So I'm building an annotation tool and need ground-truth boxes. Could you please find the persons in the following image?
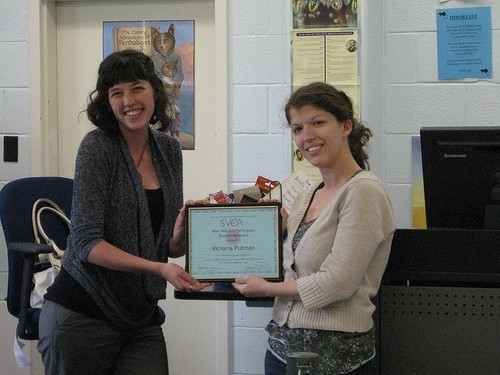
[37,49,213,375]
[234,81,397,375]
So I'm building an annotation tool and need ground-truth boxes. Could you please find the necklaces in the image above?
[133,139,149,168]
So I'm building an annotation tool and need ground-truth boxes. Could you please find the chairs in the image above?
[0,176,74,341]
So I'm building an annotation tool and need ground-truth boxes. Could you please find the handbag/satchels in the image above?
[27,198,73,310]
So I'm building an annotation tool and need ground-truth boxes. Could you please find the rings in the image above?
[189,285,193,291]
